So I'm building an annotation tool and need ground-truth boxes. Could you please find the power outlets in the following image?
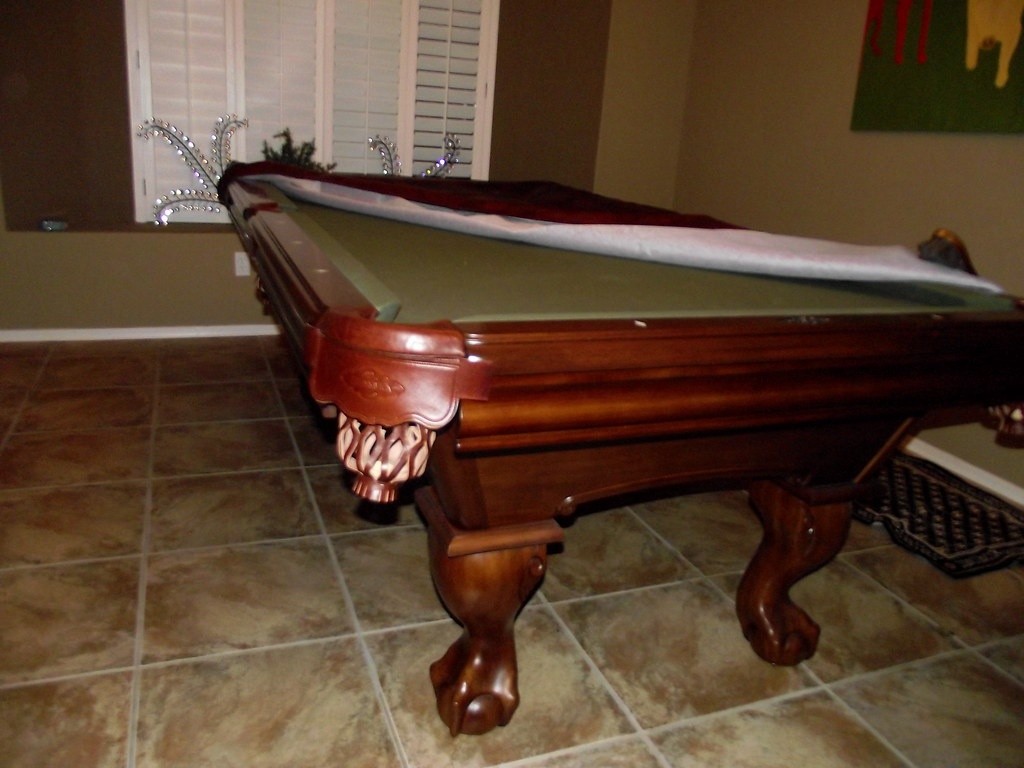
[233,252,252,277]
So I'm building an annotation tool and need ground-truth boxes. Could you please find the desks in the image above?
[215,161,1024,739]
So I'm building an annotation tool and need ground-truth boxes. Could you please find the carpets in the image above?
[847,449,1024,582]
